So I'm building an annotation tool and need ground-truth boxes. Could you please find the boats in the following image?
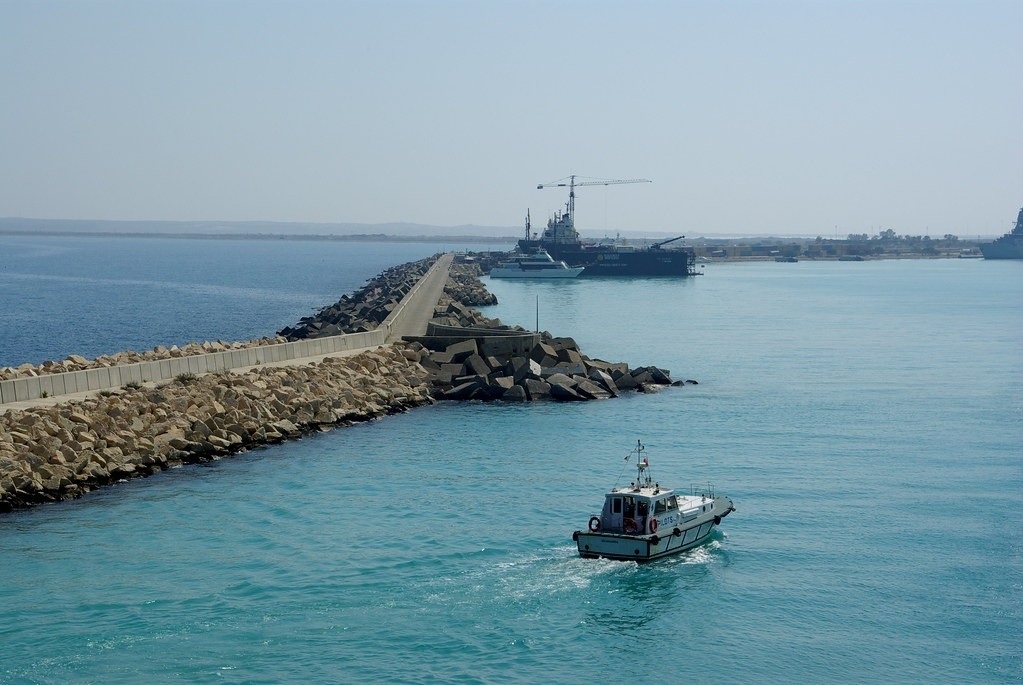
[572,438,738,564]
[518,199,689,276]
[488,207,586,279]
[978,206,1022,260]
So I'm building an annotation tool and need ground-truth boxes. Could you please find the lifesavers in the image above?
[648,517,659,534]
[588,516,601,532]
[622,518,637,534]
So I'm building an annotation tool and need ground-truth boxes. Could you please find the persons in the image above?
[702,494,705,502]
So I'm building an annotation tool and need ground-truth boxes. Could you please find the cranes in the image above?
[536,174,655,227]
